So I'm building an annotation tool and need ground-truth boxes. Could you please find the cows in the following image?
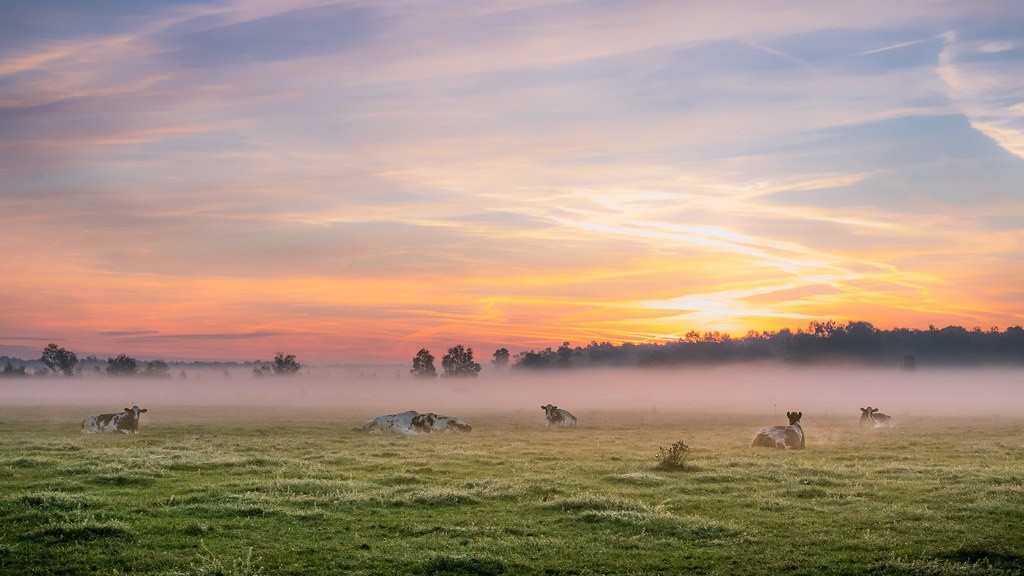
[80,405,148,435]
[541,404,577,427]
[859,407,895,429]
[749,412,806,450]
[352,411,472,437]
